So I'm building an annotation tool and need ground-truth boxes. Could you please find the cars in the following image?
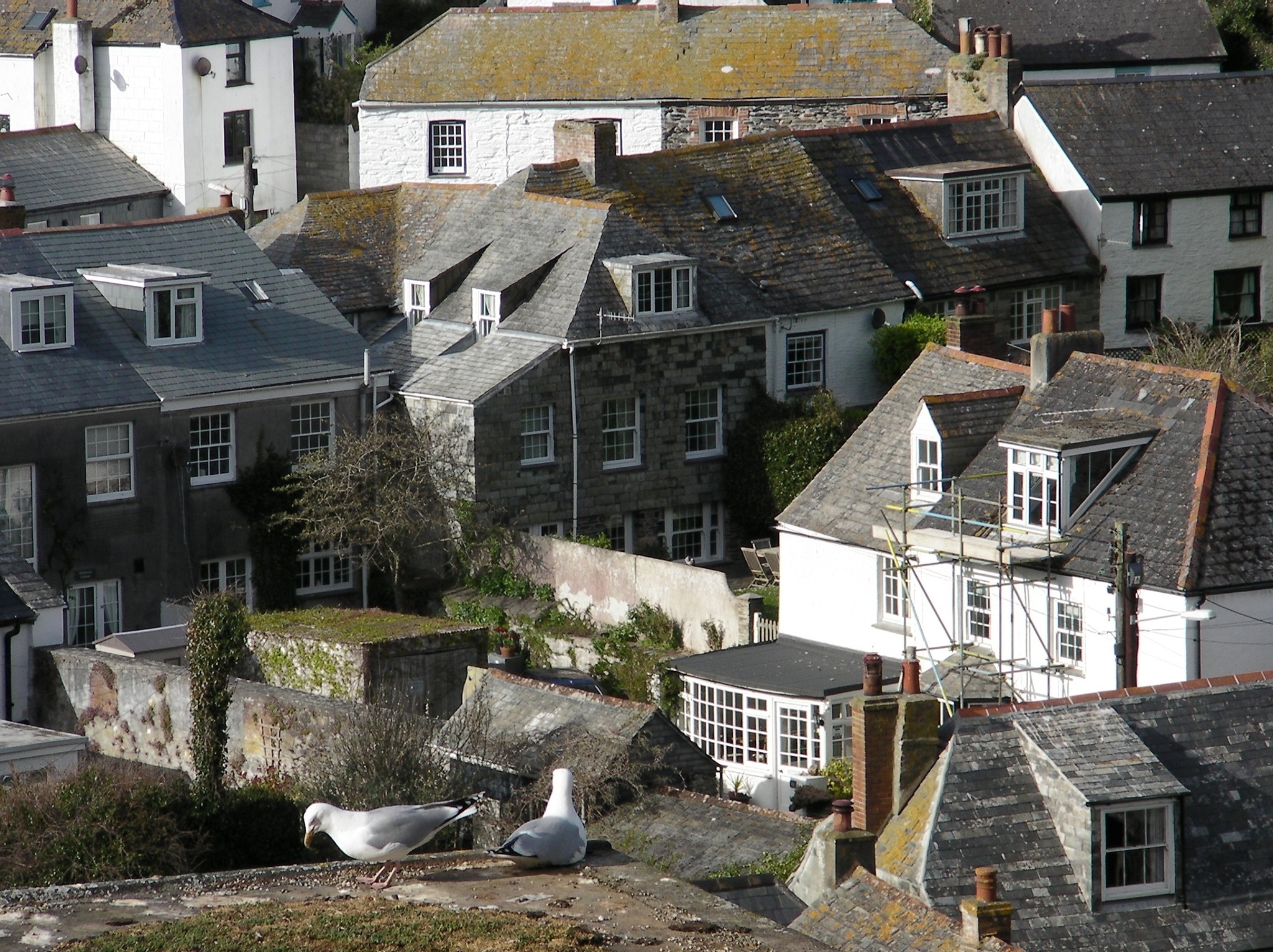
[517,668,605,698]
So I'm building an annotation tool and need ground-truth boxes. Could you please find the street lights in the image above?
[1125,608,1218,688]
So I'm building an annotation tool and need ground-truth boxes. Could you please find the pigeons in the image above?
[492,767,588,868]
[302,795,490,887]
